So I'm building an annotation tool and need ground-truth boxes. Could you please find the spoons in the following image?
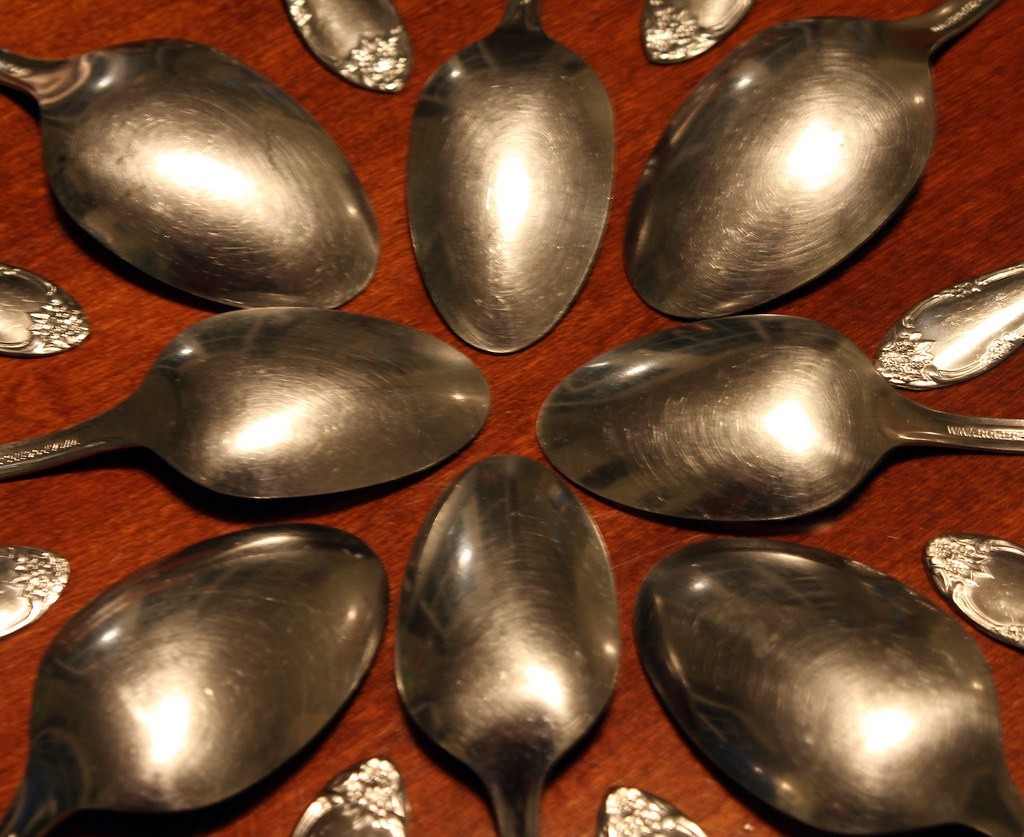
[0,37,382,311]
[403,1,614,356]
[0,306,492,500]
[534,313,1024,523]
[633,535,1024,837]
[625,0,1006,320]
[1,522,387,836]
[393,454,623,837]
